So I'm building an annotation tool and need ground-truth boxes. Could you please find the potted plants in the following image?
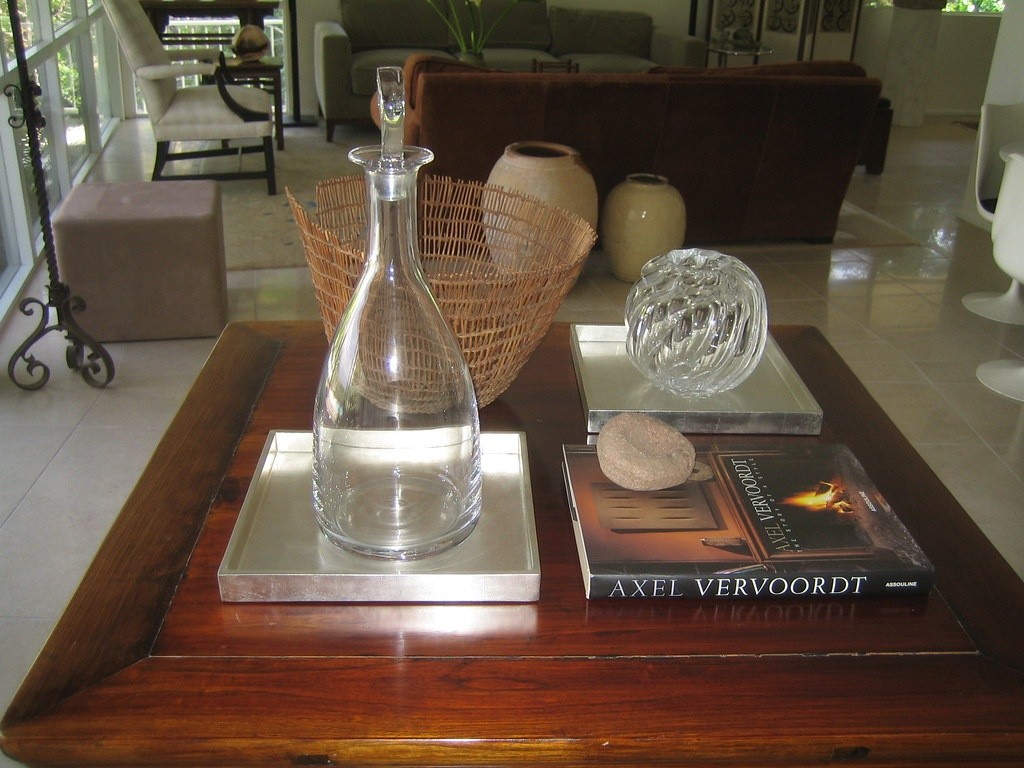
[426,0,518,67]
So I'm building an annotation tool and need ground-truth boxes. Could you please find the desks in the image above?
[707,45,775,68]
[138,0,279,88]
[0,321,1024,768]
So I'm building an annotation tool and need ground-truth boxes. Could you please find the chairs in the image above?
[102,0,277,195]
[961,103,1024,400]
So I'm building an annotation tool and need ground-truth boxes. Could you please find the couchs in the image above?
[314,0,708,153]
[365,54,882,245]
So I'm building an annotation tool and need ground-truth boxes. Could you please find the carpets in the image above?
[146,136,928,269]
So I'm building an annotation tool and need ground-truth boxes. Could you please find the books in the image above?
[561,444,935,600]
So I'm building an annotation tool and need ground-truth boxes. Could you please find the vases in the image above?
[482,139,599,299]
[602,174,686,285]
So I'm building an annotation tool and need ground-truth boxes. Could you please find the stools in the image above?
[48,179,227,345]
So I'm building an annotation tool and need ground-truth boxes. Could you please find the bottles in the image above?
[484,139,601,299]
[599,172,687,286]
[310,66,486,563]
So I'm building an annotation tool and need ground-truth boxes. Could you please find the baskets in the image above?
[284,173,599,414]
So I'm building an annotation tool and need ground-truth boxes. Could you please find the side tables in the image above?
[200,54,286,153]
